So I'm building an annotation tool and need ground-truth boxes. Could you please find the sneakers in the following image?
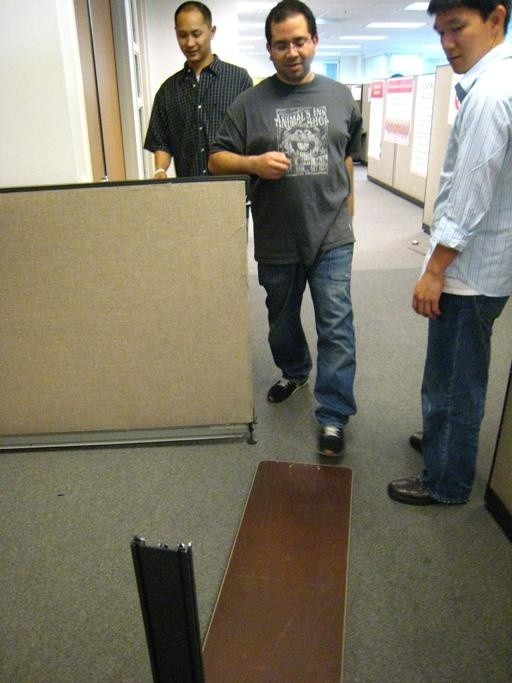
[317,425,344,457]
[267,375,310,403]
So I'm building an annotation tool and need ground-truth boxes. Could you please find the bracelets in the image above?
[153,168,165,176]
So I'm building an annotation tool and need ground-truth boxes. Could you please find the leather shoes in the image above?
[389,477,438,506]
[410,432,424,451]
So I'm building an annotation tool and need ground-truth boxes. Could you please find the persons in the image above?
[206,0,363,458]
[143,0,253,247]
[388,0,510,506]
